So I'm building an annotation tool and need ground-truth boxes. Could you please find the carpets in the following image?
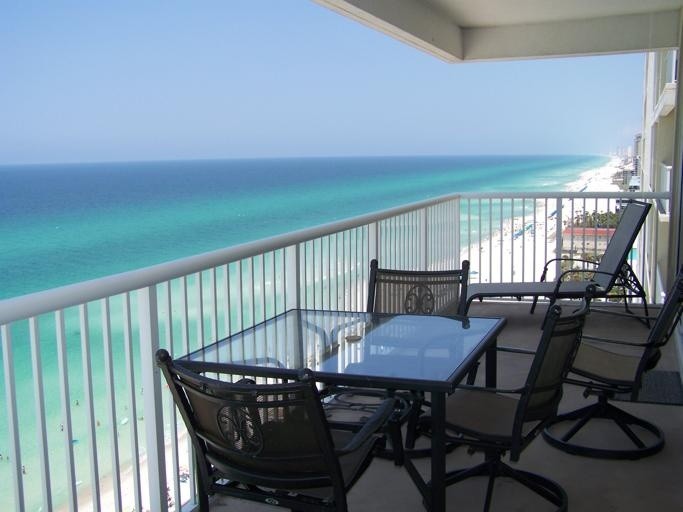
[606,370,681,407]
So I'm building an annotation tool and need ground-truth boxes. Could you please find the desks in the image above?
[182,306,510,511]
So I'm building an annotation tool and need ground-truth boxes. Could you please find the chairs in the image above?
[411,281,595,511]
[460,197,651,327]
[151,339,388,511]
[350,256,471,446]
[543,251,682,458]
